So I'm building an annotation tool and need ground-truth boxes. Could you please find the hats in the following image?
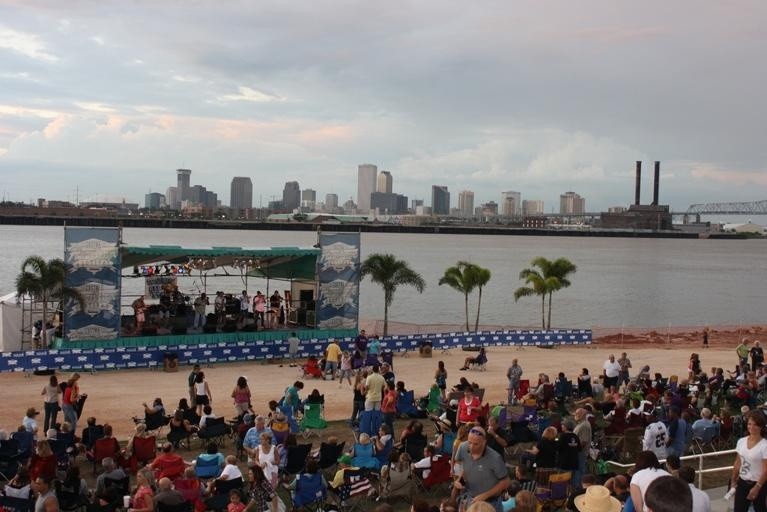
[375,503,394,512]
[46,320,52,329]
[337,455,353,465]
[26,408,40,418]
[273,414,288,424]
[625,408,642,420]
[436,419,452,432]
[257,291,262,294]
[553,433,586,470]
[34,320,41,331]
[638,400,656,416]
[745,371,757,378]
[66,465,82,481]
[271,422,288,433]
[741,405,750,413]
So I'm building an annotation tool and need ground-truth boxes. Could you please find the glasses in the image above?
[470,427,486,440]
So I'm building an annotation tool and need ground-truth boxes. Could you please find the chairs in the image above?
[85,499,119,512]
[158,498,192,511]
[183,405,198,424]
[300,403,324,438]
[10,431,35,455]
[396,390,415,415]
[87,440,120,475]
[434,432,459,454]
[643,402,657,422]
[627,411,642,427]
[229,414,257,433]
[553,379,573,406]
[471,353,487,372]
[354,443,375,460]
[711,417,735,451]
[507,379,530,401]
[377,437,394,459]
[53,480,85,510]
[329,466,370,508]
[533,383,554,408]
[122,435,156,475]
[604,407,626,436]
[306,394,325,404]
[371,471,412,505]
[573,377,591,395]
[133,407,165,436]
[282,472,325,512]
[5,482,33,500]
[687,422,722,455]
[309,440,347,469]
[208,476,243,506]
[392,433,428,458]
[198,417,225,449]
[623,426,642,464]
[194,456,221,478]
[279,442,313,474]
[510,420,530,437]
[82,425,105,457]
[412,452,451,498]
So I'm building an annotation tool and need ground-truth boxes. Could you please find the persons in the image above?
[127,468,154,512]
[541,499,557,511]
[485,416,510,465]
[642,407,667,469]
[126,422,156,454]
[94,423,121,453]
[366,365,374,375]
[282,460,328,504]
[396,380,408,396]
[254,432,281,490]
[350,349,364,370]
[361,368,368,385]
[59,466,89,511]
[375,502,395,511]
[13,425,34,460]
[61,379,78,433]
[532,372,545,392]
[470,382,480,392]
[31,322,41,351]
[306,355,327,380]
[603,352,690,423]
[215,290,228,333]
[409,499,431,512]
[254,291,265,329]
[68,372,82,410]
[145,441,184,478]
[276,434,297,468]
[572,407,592,493]
[437,398,459,434]
[336,354,342,370]
[271,413,289,433]
[45,428,67,461]
[198,405,220,430]
[439,496,458,512]
[629,450,711,511]
[0,427,18,464]
[458,347,485,370]
[270,290,284,330]
[231,376,256,420]
[577,367,592,383]
[557,416,581,471]
[310,436,338,463]
[521,426,558,468]
[400,417,428,449]
[566,474,587,512]
[73,447,89,464]
[242,415,278,463]
[430,505,439,512]
[591,375,605,402]
[141,264,187,275]
[41,375,62,437]
[214,454,246,483]
[429,418,455,453]
[603,474,630,508]
[170,285,187,311]
[453,376,476,394]
[169,407,193,450]
[192,442,226,469]
[380,451,412,499]
[501,479,522,511]
[226,488,246,512]
[454,425,514,512]
[268,400,280,418]
[354,329,369,361]
[664,454,682,476]
[131,294,150,337]
[40,321,62,349]
[665,404,689,461]
[22,406,41,446]
[410,446,452,481]
[328,454,361,496]
[521,375,551,401]
[367,332,382,361]
[363,365,391,412]
[300,389,325,405]
[514,463,538,493]
[188,363,201,408]
[287,332,300,367]
[349,373,367,428]
[81,416,104,450]
[2,465,38,500]
[456,386,483,429]
[234,290,256,310]
[553,371,568,403]
[336,349,353,391]
[30,440,57,482]
[731,409,767,512]
[465,500,496,512]
[644,474,694,512]
[574,396,616,416]
[142,397,166,419]
[506,358,523,407]
[509,489,540,512]
[193,292,210,331]
[93,456,128,497]
[316,351,333,375]
[193,371,212,417]
[369,424,393,457]
[379,377,397,441]
[321,338,342,381]
[242,465,287,512]
[688,325,767,512]
[59,422,75,449]
[380,361,395,404]
[177,465,216,492]
[152,477,185,512]
[178,398,200,425]
[284,381,304,418]
[414,383,439,413]
[158,288,171,320]
[434,360,448,403]
[345,432,377,469]
[34,475,59,512]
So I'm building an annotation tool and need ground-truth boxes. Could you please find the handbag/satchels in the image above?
[264,496,286,512]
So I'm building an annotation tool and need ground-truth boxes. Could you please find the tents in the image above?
[119,243,320,279]
